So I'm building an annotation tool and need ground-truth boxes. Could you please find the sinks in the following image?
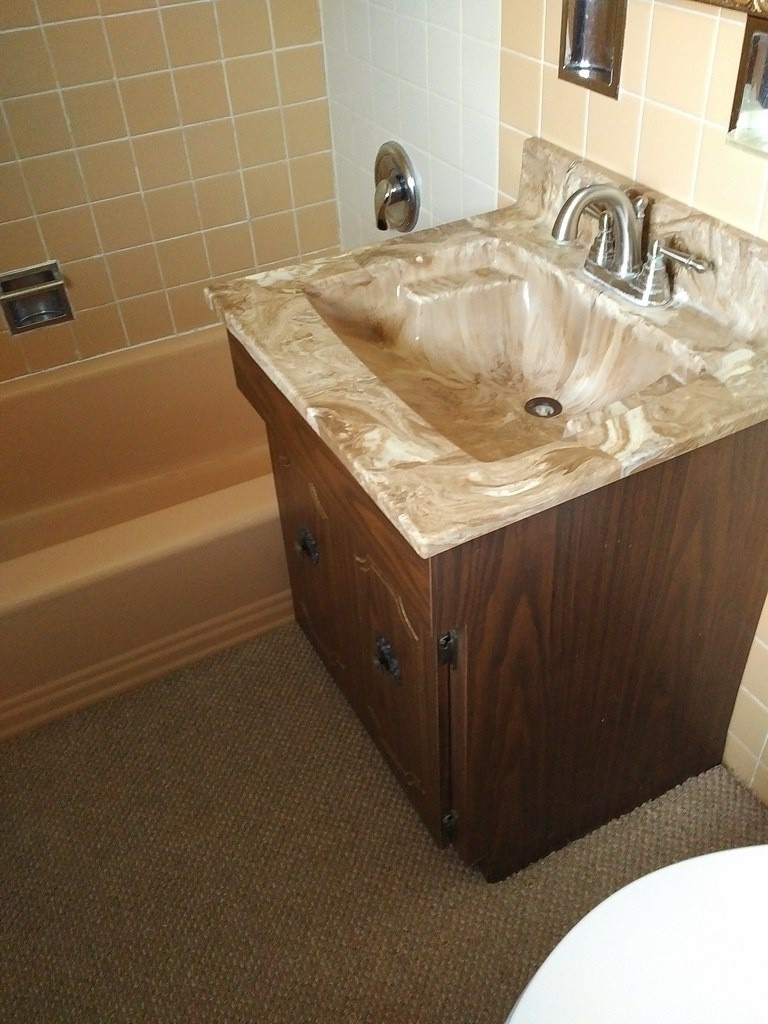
[199,135,768,564]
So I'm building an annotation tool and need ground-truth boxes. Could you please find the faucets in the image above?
[550,181,645,285]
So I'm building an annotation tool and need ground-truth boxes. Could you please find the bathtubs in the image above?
[0,321,294,733]
